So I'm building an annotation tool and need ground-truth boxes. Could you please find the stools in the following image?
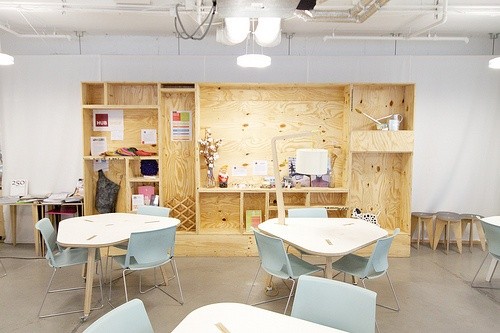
[412,211,486,254]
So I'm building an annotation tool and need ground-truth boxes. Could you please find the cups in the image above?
[388,119,400,132]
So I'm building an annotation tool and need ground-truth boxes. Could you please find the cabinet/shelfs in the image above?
[80,81,416,257]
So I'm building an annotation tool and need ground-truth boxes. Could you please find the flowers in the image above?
[199,128,223,178]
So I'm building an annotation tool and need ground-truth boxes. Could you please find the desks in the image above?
[0,197,83,259]
[57,213,181,322]
[170,302,349,333]
[259,218,389,280]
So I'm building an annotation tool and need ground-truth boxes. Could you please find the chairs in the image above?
[35,205,184,333]
[471,216,500,288]
[251,207,400,333]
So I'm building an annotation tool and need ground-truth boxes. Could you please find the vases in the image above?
[205,167,216,188]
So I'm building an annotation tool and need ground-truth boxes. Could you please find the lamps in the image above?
[296,149,329,187]
[216,17,282,68]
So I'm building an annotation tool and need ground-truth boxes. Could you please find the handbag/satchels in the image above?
[140,160,158,176]
[291,173,310,187]
[95,169,120,214]
